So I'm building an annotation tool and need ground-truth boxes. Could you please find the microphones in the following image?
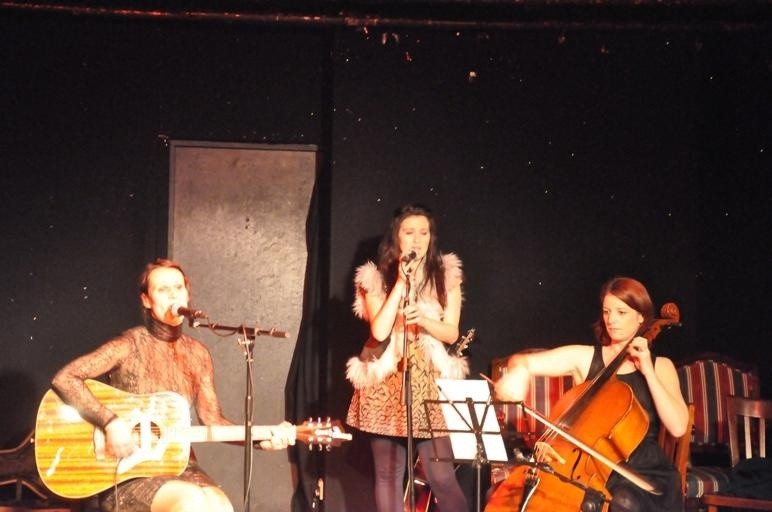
[170,297,205,322]
[403,249,416,266]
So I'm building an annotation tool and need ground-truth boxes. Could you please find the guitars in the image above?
[33,377,352,499]
[404,328,475,511]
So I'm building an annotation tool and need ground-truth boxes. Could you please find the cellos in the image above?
[480,303,680,512]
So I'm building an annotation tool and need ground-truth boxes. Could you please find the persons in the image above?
[343,202,467,511]
[493,276,690,512]
[49,257,297,512]
